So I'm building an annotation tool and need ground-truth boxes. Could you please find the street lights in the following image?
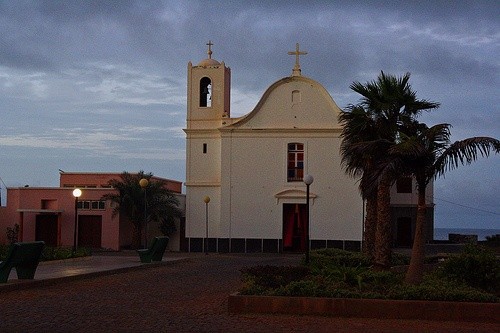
[140,179,149,255]
[204,196,211,254]
[301,174,314,264]
[71,189,83,250]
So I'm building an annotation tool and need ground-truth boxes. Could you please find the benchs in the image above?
[136,236,170,264]
[0,241,45,283]
